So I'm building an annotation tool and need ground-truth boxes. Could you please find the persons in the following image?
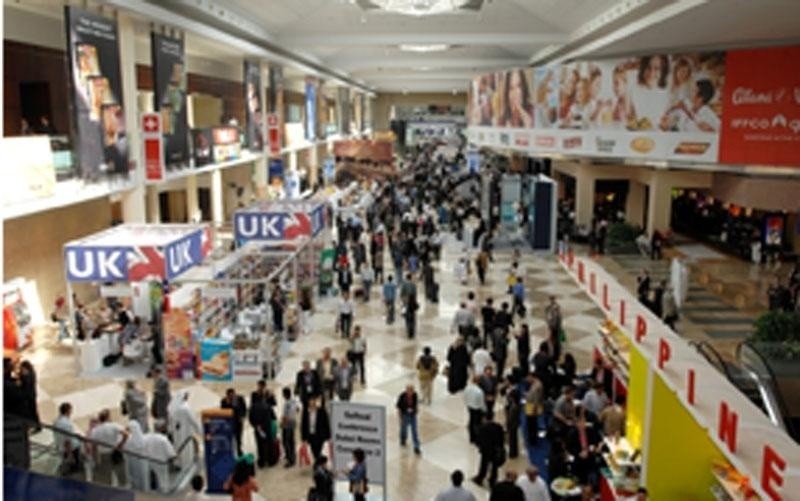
[476,53,721,131]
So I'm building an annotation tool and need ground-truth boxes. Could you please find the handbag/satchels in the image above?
[349,477,368,495]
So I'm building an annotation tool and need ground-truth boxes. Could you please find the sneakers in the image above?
[400,439,421,456]
[471,476,496,488]
[341,332,350,338]
[418,399,432,406]
[254,456,297,469]
[360,380,366,385]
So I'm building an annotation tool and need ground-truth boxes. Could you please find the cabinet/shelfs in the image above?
[160,281,240,376]
[265,250,314,315]
[216,253,265,316]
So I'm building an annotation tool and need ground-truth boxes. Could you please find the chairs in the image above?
[44,303,128,373]
